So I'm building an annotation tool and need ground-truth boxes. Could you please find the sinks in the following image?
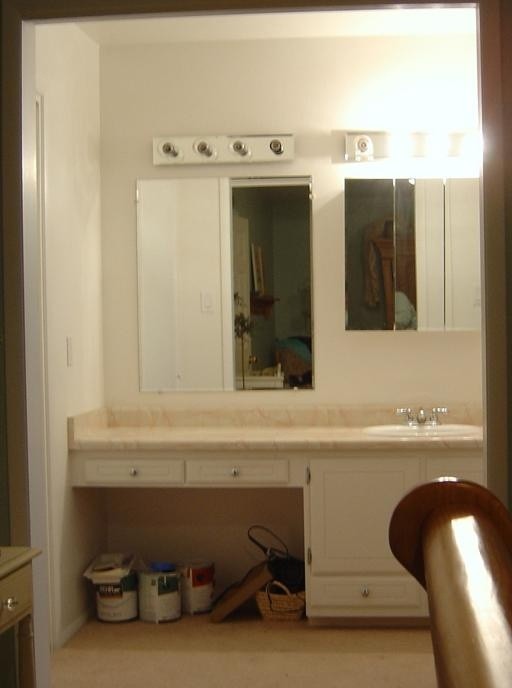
[363,424,482,437]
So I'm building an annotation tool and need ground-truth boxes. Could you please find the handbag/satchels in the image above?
[268,555,305,586]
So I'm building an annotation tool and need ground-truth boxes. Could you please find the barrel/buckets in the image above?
[137,569,182,624]
[96,571,139,623]
[176,560,215,616]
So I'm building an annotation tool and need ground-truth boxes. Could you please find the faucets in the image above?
[416,407,426,424]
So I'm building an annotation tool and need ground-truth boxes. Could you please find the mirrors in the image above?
[345,178,486,331]
[135,175,315,392]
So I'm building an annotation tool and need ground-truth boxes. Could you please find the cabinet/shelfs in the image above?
[68,452,309,487]
[0,546,42,688]
[304,450,483,627]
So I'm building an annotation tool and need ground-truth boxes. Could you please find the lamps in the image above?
[344,129,388,161]
[152,134,293,167]
